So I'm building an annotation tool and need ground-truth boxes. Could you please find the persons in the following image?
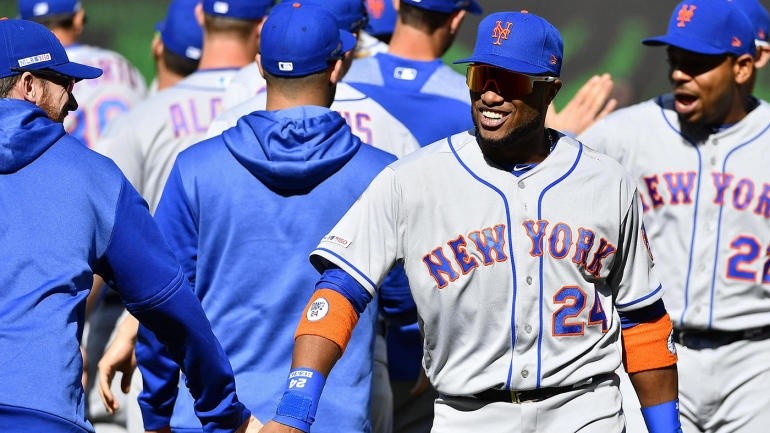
[252,9,686,433]
[0,0,769,433]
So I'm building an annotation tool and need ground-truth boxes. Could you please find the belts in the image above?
[672,325,770,349]
[438,371,615,404]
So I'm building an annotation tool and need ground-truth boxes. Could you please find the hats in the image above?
[260,1,356,77]
[641,0,756,58]
[400,0,482,14]
[728,0,770,41]
[17,0,82,19]
[154,0,203,62]
[0,19,103,83]
[362,0,398,36]
[281,0,368,32]
[452,10,563,77]
[203,0,274,20]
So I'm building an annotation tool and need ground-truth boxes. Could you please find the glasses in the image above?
[465,64,558,99]
[11,67,75,95]
